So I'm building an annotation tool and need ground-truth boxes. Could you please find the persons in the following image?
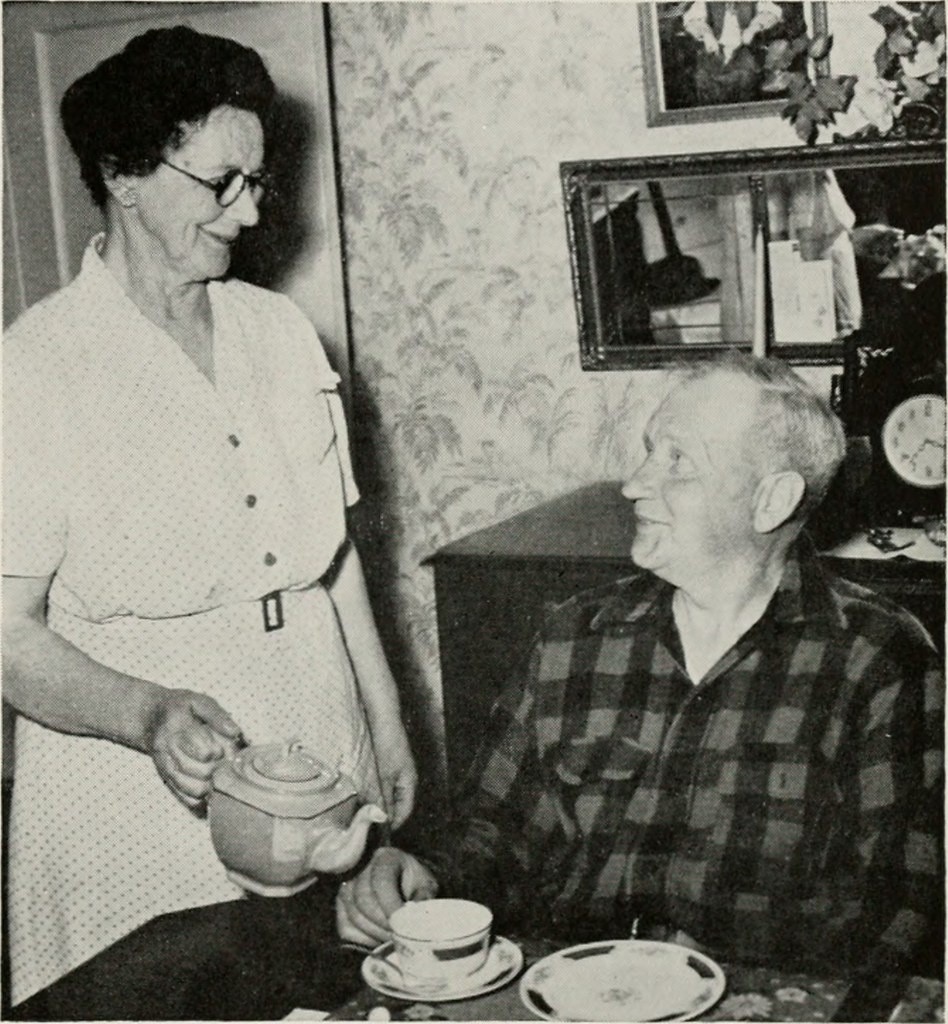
[2,23,419,1022]
[335,353,945,976]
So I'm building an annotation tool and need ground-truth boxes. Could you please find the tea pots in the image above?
[202,723,389,899]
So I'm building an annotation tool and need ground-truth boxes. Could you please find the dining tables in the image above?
[5,889,944,1023]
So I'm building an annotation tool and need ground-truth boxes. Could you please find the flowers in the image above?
[779,2,944,149]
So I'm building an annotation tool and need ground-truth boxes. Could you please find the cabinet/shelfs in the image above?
[418,478,945,798]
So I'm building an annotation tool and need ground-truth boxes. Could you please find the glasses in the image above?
[159,155,278,208]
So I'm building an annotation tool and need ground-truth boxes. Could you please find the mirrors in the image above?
[559,137,945,371]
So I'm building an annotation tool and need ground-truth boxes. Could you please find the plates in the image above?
[360,935,524,1002]
[518,939,727,1022]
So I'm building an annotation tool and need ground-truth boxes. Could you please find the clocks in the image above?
[828,269,944,524]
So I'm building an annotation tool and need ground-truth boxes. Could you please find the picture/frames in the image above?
[637,3,832,128]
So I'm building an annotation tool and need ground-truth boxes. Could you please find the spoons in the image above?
[340,944,449,993]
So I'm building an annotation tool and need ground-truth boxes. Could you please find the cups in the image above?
[388,898,493,980]
